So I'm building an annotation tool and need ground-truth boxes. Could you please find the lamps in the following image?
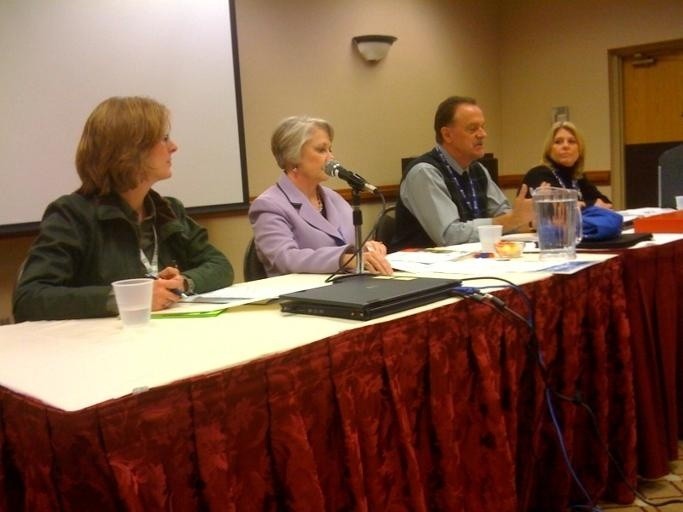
[352,33,398,63]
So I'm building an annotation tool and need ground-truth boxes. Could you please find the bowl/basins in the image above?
[495,241,524,257]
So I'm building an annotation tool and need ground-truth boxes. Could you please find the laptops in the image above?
[278,274,462,322]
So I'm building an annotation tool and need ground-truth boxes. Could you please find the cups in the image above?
[478,225,503,252]
[674,195,683,210]
[530,186,584,260]
[111,278,155,327]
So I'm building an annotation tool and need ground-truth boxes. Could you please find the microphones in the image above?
[320,159,378,196]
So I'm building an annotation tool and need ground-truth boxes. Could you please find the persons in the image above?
[11,96,234,321]
[515,119,615,233]
[386,97,551,254]
[245,117,396,278]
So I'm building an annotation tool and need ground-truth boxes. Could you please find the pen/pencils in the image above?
[145,273,188,299]
